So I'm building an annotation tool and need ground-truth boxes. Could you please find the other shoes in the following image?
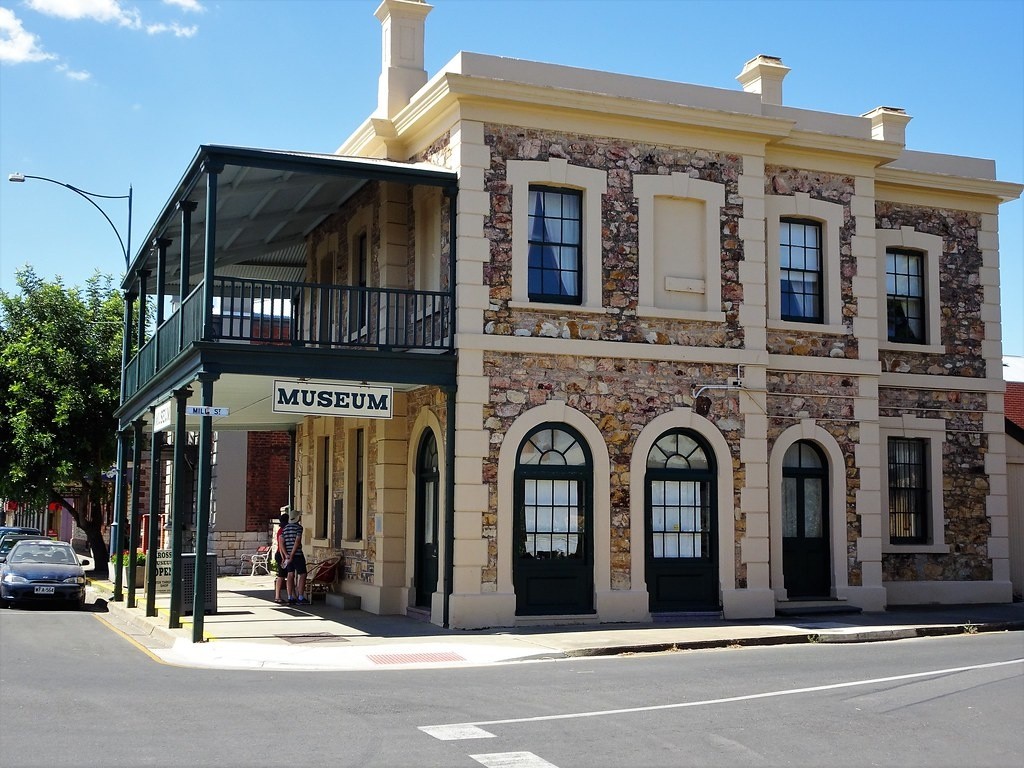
[296,597,312,605]
[287,598,296,606]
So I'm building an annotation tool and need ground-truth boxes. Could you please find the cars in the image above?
[0,527,90,609]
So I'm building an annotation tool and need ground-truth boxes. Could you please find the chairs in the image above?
[296,557,340,606]
[238,546,273,576]
[51,551,65,562]
[20,552,34,561]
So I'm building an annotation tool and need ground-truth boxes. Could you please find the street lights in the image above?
[7,172,133,560]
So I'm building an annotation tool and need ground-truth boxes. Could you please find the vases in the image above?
[108,562,145,588]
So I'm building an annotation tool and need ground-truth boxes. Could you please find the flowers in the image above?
[110,550,146,566]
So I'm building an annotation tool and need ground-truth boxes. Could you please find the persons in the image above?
[275,510,313,605]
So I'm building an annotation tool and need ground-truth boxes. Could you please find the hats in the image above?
[288,510,304,523]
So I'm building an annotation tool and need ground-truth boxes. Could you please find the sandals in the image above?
[275,599,286,603]
